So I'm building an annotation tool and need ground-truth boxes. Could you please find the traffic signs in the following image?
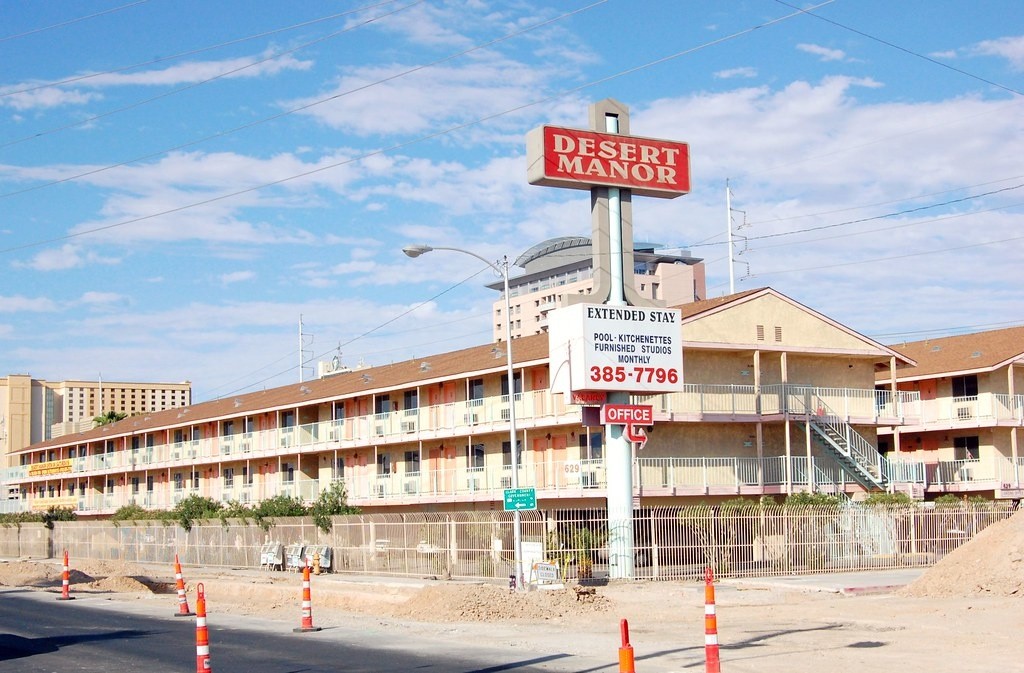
[503,487,537,512]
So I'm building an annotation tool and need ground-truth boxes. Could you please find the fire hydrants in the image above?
[311,552,321,575]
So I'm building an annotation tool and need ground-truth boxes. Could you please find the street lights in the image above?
[401,244,527,591]
